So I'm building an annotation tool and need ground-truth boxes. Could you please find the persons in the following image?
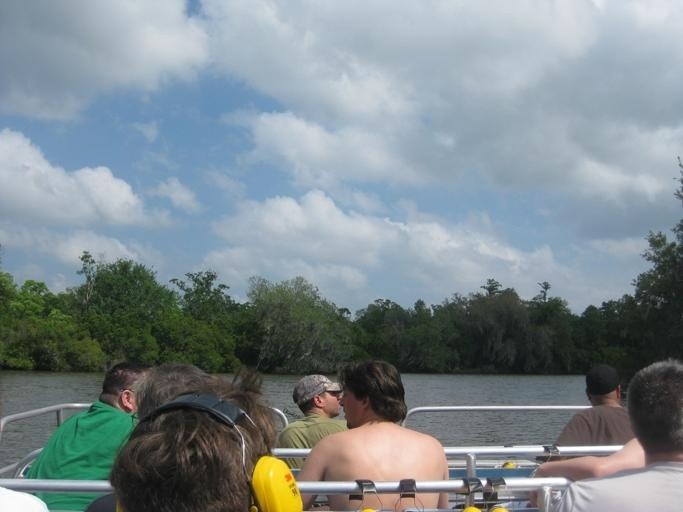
[526,435,647,479]
[551,359,683,512]
[19,358,452,512]
[549,364,637,460]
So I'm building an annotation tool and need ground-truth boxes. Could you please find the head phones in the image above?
[112,390,305,512]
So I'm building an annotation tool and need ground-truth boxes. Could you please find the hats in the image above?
[293,373,344,407]
[586,364,619,395]
